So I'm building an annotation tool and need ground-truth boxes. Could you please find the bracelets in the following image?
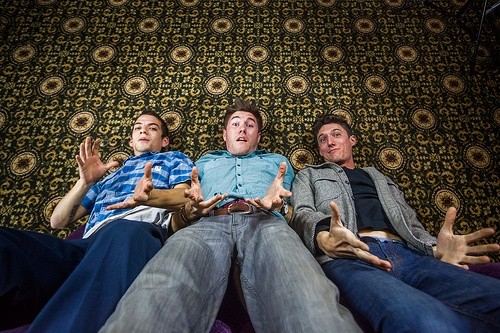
[179,207,193,225]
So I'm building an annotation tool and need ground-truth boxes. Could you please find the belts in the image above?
[204,203,263,217]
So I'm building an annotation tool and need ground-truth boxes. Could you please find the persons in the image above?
[98,99,364,333]
[0,109,201,333]
[288,114,500,333]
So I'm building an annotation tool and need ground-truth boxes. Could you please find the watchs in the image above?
[279,202,288,216]
